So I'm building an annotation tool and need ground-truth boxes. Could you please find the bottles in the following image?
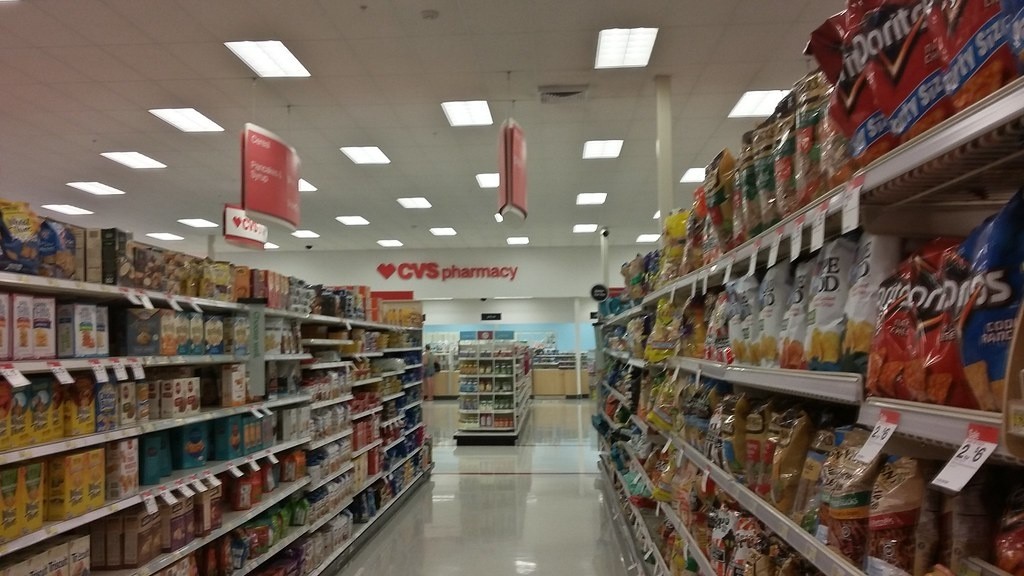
[459,380,512,393]
[459,362,513,376]
[460,399,512,410]
[458,415,514,427]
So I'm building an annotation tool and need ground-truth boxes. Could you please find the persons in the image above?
[422,344,435,401]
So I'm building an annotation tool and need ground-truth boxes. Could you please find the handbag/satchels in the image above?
[434,362,441,372]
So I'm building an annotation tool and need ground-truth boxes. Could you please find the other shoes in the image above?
[425,397,433,401]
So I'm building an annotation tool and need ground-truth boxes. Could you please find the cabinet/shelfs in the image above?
[0,265,532,576]
[529,347,566,398]
[588,71,1024,576]
[559,352,589,400]
